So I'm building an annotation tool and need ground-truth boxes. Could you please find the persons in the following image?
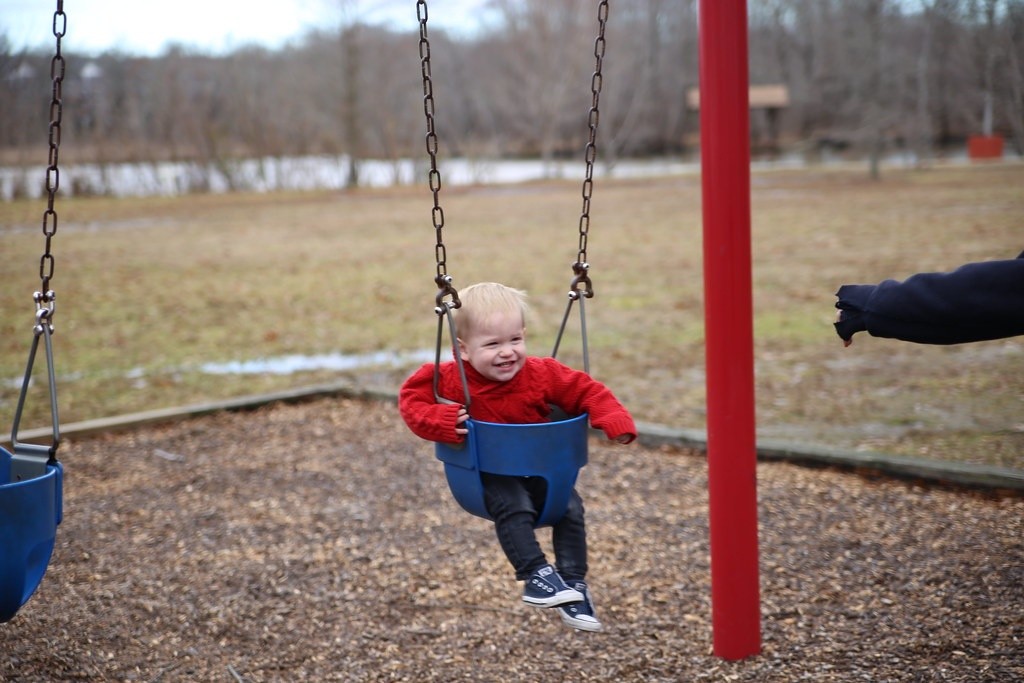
[834,249,1024,348]
[398,282,638,632]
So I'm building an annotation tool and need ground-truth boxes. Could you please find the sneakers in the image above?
[521,564,585,608]
[555,579,604,633]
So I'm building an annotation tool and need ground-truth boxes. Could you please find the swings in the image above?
[0,0,68,625]
[412,0,613,527]
